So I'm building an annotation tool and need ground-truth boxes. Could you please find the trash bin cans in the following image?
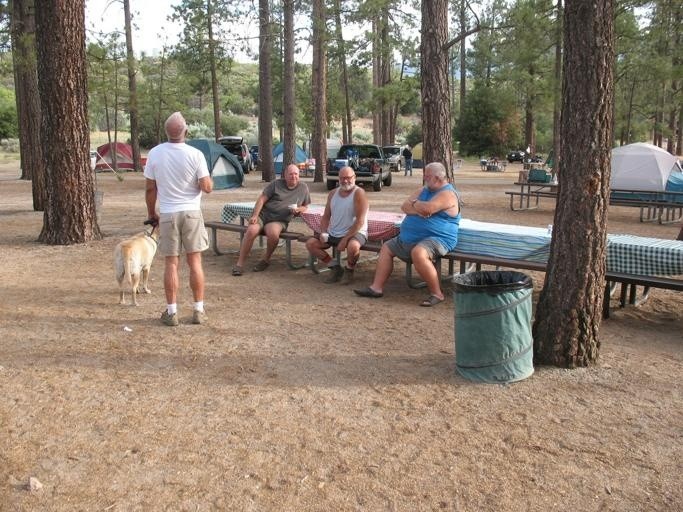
[451,270,535,384]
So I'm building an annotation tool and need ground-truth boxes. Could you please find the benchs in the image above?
[202,221,683,318]
[505,191,683,224]
[531,191,683,207]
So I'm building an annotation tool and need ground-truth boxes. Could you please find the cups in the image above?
[322,233,329,242]
[287,203,297,209]
[545,174,552,183]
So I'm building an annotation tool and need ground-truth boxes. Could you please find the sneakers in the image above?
[231,265,242,277]
[339,266,354,285]
[250,259,270,272]
[159,308,179,327]
[322,264,344,283]
[191,306,209,324]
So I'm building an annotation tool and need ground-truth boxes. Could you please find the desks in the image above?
[513,182,683,221]
[222,202,683,308]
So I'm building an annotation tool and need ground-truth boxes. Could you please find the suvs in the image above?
[219,135,254,173]
[382,144,413,174]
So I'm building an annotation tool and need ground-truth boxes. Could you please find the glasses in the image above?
[339,174,354,179]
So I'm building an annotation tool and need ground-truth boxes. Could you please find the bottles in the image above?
[546,225,553,238]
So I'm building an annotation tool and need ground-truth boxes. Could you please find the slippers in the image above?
[352,286,384,298]
[418,295,445,308]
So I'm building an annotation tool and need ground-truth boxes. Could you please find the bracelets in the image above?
[411,199,417,207]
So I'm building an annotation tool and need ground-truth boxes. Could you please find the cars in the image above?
[506,149,542,164]
[249,143,258,161]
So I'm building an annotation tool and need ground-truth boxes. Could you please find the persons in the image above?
[229,164,311,276]
[354,161,461,308]
[143,112,214,325]
[303,165,369,284]
[402,145,412,177]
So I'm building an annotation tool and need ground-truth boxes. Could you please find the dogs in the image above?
[113,223,161,307]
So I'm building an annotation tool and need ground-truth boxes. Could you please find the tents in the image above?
[256,138,306,174]
[95,143,144,171]
[185,138,245,191]
[608,142,682,204]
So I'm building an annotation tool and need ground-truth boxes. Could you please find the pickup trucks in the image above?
[325,142,393,191]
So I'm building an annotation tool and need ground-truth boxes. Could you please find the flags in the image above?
[302,138,342,159]
